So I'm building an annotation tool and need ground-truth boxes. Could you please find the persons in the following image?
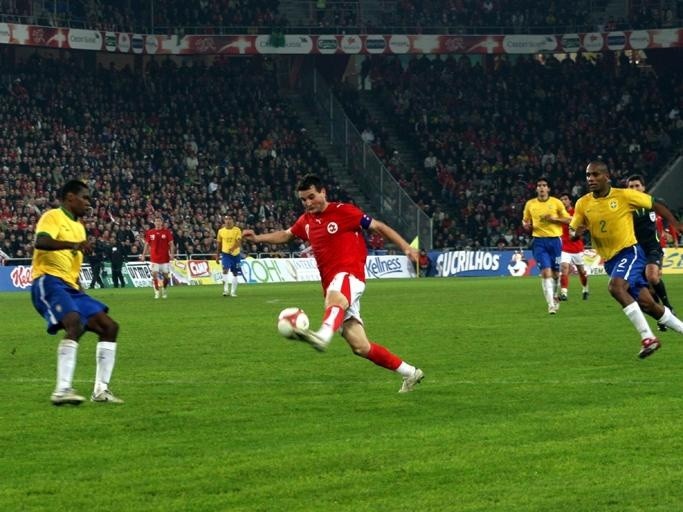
[608,178,624,188]
[521,50,682,178]
[216,215,242,297]
[522,178,537,205]
[29,179,124,405]
[572,178,588,206]
[335,55,425,173]
[568,160,683,358]
[242,173,425,394]
[232,217,242,229]
[216,217,224,230]
[305,1,682,34]
[0,56,29,257]
[175,217,216,260]
[242,56,335,173]
[140,217,175,255]
[124,217,140,260]
[1,1,152,35]
[29,179,69,254]
[624,174,683,333]
[140,217,175,300]
[522,178,572,314]
[556,193,589,301]
[29,56,124,179]
[242,173,310,258]
[415,249,431,277]
[124,56,242,217]
[425,51,521,248]
[80,179,124,290]
[522,228,533,250]
[663,207,683,248]
[548,178,572,199]
[320,173,425,249]
[154,2,291,36]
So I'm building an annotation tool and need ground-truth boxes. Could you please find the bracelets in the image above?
[74,243,79,250]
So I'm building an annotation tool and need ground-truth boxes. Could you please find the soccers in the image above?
[278,307,309,340]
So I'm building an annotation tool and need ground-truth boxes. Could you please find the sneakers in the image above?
[583,291,589,300]
[232,294,238,297]
[162,293,167,298]
[154,293,159,299]
[558,294,568,301]
[294,328,328,353]
[223,291,230,296]
[657,304,675,332]
[50,387,86,402]
[548,294,559,314]
[91,388,124,404]
[639,336,661,359]
[398,368,424,394]
